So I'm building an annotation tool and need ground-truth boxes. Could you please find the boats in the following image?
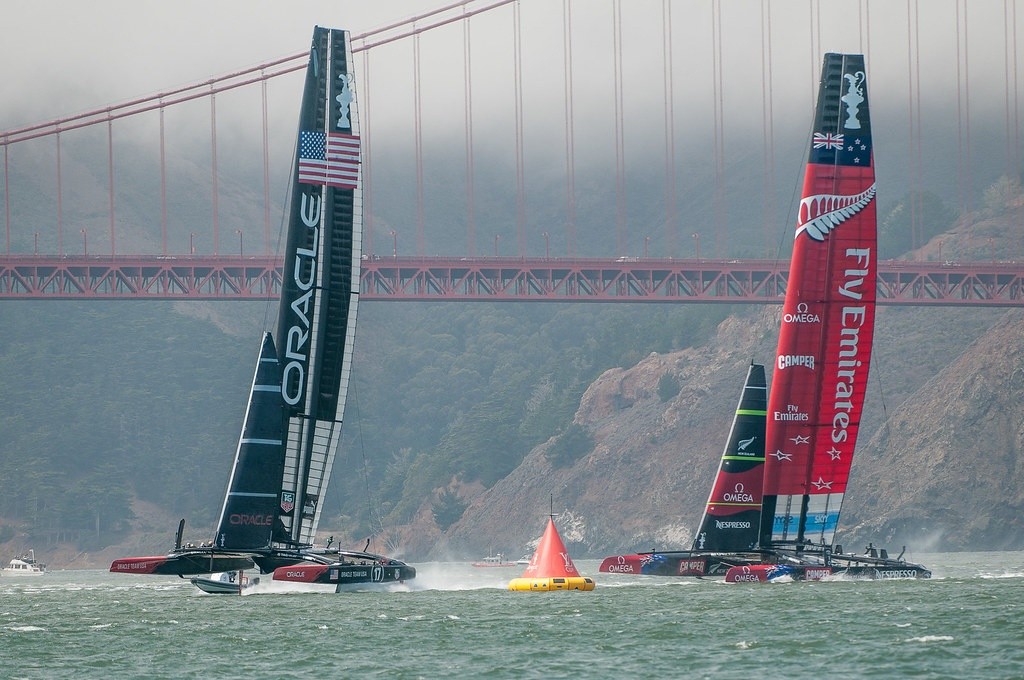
[471,552,515,567]
[518,554,533,564]
[2,549,47,578]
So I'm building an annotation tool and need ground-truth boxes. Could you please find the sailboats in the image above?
[108,24,416,594]
[599,52,936,583]
